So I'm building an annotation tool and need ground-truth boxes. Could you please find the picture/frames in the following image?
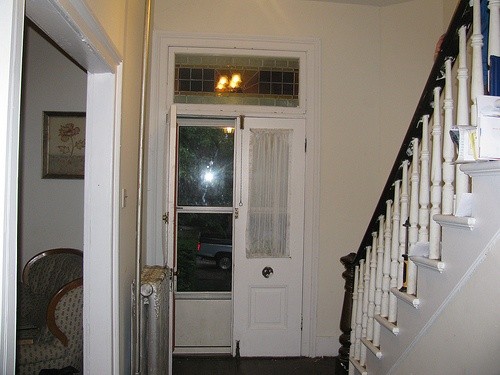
[41,110,86,179]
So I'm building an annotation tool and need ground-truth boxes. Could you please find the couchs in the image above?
[14,247,83,375]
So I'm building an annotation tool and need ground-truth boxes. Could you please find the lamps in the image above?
[214,71,243,90]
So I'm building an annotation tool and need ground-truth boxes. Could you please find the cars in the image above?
[197,224,231,270]
